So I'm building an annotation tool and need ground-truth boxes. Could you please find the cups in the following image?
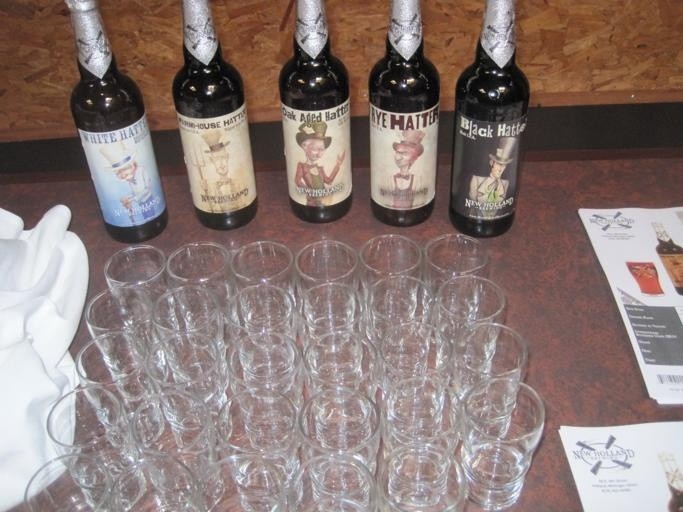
[25,234,548,512]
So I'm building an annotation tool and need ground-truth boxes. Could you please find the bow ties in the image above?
[309,163,319,169]
[396,172,411,180]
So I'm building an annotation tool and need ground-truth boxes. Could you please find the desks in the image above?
[7,151,682,512]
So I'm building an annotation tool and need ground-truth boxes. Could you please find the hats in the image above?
[202,132,230,153]
[296,121,331,148]
[100,143,136,172]
[489,137,515,164]
[393,129,424,155]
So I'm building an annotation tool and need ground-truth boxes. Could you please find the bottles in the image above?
[64,0,170,244]
[448,0,532,239]
[276,0,355,224]
[170,4,258,232]
[367,1,440,227]
